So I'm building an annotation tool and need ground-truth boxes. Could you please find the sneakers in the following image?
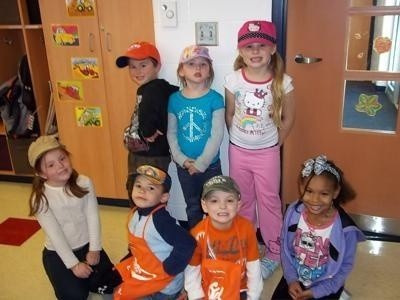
[260,253,282,279]
[339,288,351,300]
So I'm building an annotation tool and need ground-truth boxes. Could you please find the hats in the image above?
[127,164,173,193]
[179,45,213,65]
[28,135,62,168]
[237,19,277,47]
[115,41,161,70]
[201,174,241,203]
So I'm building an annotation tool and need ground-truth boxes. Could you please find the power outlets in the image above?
[195,21,219,47]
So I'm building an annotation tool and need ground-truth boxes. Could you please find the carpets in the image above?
[0,217,41,247]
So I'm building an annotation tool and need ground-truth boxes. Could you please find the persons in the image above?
[113,164,197,300]
[164,44,227,223]
[220,19,295,261]
[25,132,120,300]
[183,177,265,300]
[110,40,181,177]
[270,156,358,299]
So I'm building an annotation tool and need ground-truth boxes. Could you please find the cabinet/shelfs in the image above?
[0,0,157,207]
